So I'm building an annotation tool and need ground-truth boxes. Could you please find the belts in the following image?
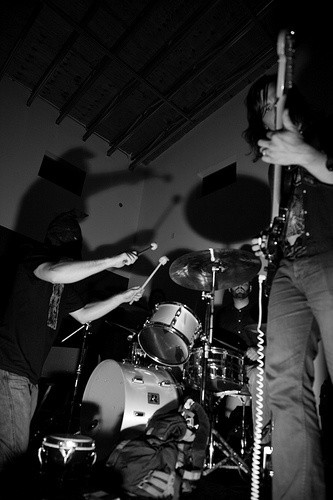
[280,245,309,257]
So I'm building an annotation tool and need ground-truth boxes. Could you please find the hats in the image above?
[48,214,81,228]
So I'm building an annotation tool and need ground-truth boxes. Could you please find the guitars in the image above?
[262,30,295,296]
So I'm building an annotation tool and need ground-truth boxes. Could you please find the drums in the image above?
[138,301,202,367]
[182,347,244,393]
[79,359,182,460]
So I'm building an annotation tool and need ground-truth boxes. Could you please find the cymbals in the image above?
[169,249,263,292]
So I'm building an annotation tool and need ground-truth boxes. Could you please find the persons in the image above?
[240,72,333,500]
[214,279,256,352]
[0,225,145,473]
